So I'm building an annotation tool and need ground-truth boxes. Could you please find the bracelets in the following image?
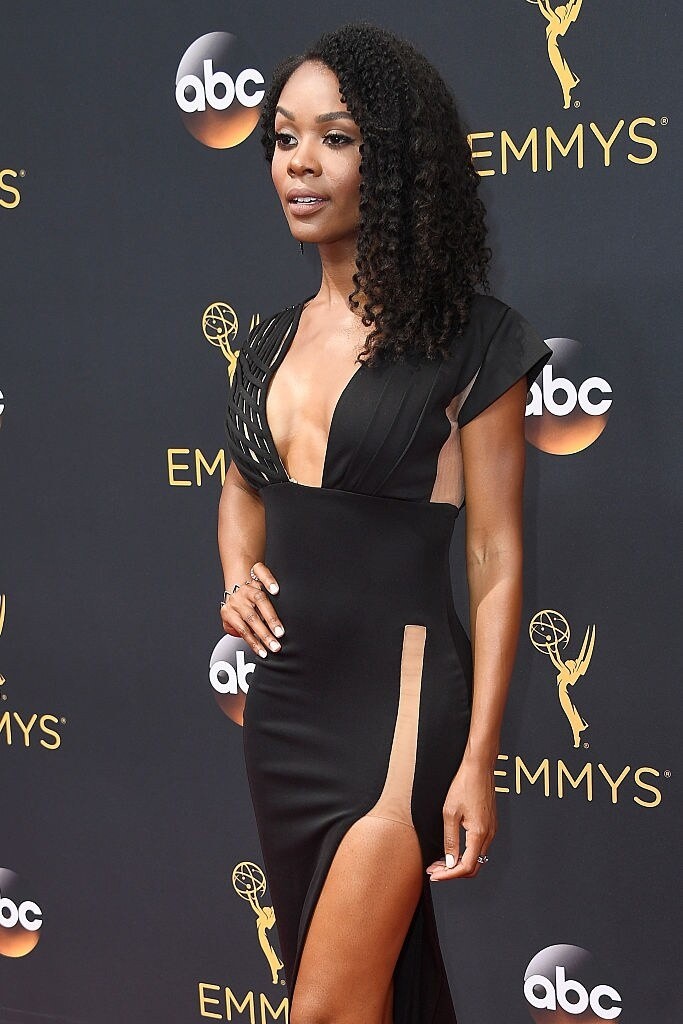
[220,580,250,608]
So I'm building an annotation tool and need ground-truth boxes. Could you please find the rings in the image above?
[249,566,262,582]
[477,854,488,865]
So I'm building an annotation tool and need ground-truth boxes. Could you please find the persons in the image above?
[216,25,556,1021]
